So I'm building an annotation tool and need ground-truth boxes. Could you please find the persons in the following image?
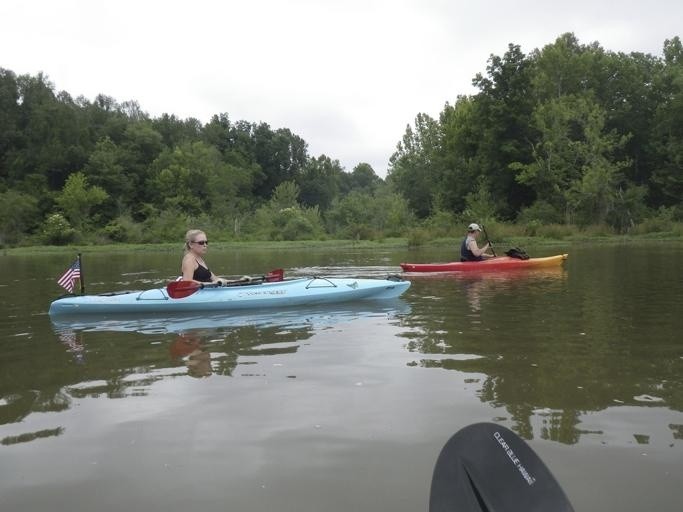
[181,229,252,288]
[464,278,481,311]
[460,223,497,262]
[187,353,212,377]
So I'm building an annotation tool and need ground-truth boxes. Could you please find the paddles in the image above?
[482,224,498,257]
[430,421,573,512]
[167,270,283,299]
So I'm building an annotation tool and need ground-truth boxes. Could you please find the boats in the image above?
[46,298,413,334]
[48,276,411,313]
[406,267,568,282]
[398,251,569,270]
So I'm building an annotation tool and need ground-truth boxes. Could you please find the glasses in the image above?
[192,241,208,245]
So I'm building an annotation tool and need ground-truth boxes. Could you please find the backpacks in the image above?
[505,246,530,260]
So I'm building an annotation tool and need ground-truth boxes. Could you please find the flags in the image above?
[57,258,81,294]
[56,330,84,364]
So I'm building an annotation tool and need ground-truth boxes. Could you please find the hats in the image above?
[468,223,482,232]
